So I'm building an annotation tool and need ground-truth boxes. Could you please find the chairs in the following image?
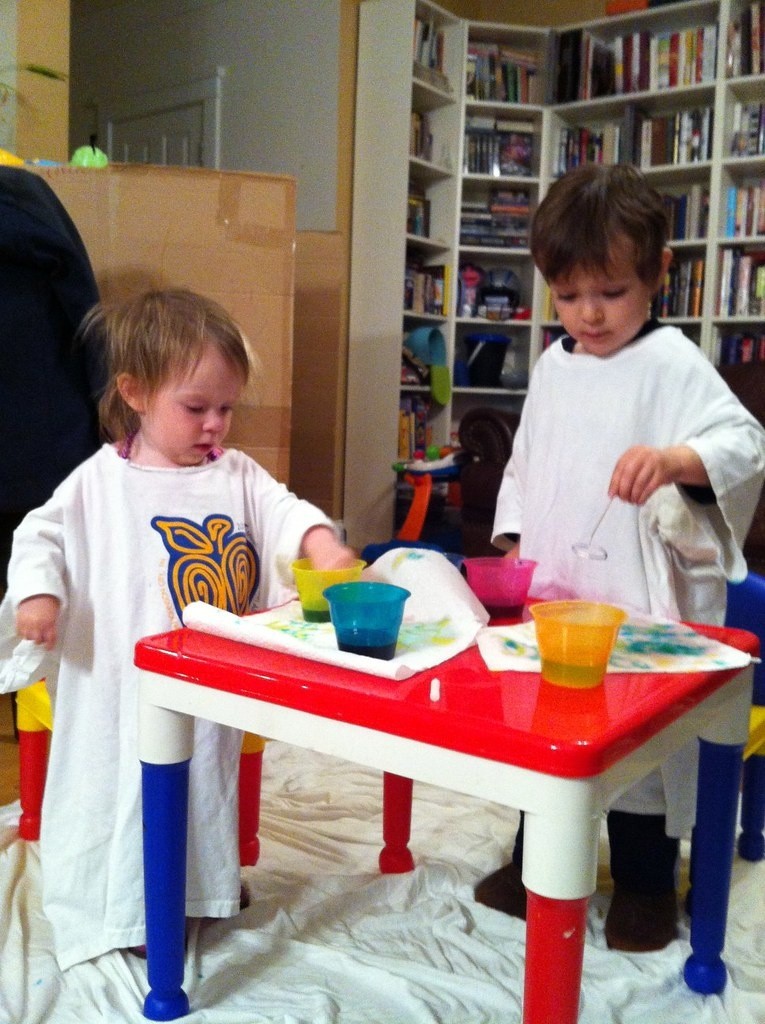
[13,676,262,869]
[723,570,765,863]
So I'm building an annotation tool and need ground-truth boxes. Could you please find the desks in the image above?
[132,595,759,1024]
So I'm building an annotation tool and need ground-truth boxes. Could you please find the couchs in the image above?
[454,359,765,577]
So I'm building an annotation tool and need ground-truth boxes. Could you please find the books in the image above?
[408,19,765,367]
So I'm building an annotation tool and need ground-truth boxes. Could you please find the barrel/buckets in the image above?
[464,332,511,387]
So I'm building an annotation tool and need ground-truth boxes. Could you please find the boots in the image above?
[603,811,682,952]
[473,810,526,922]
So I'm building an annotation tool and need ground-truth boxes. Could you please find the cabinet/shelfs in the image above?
[345,0,765,551]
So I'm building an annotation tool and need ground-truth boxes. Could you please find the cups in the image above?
[323,581,410,662]
[462,555,540,619]
[288,557,367,622]
[529,599,628,689]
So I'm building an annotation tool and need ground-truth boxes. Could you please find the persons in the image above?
[474,164,765,950]
[1,285,363,970]
